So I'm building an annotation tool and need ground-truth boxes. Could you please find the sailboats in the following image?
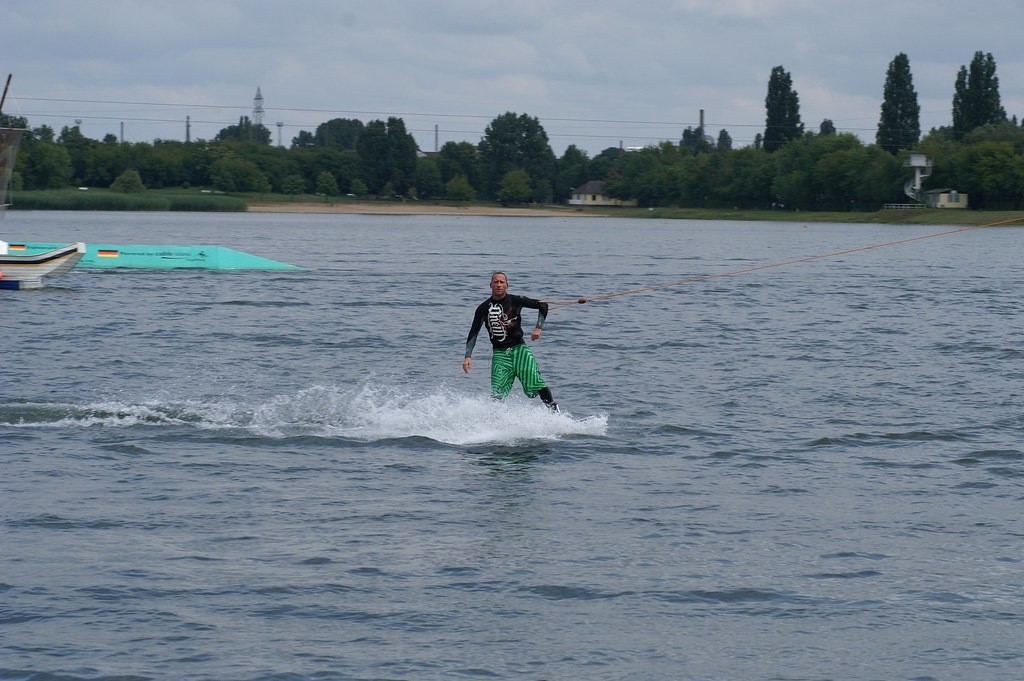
[0,73,86,291]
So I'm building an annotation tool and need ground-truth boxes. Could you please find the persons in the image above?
[461,273,558,413]
